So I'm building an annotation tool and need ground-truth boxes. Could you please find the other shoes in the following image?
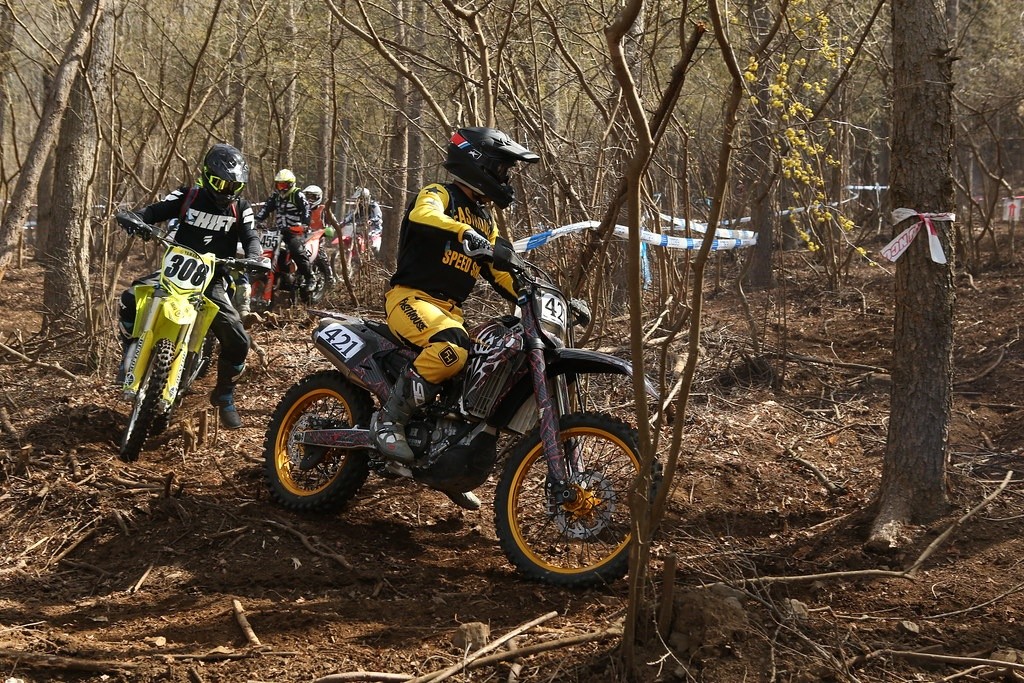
[238,310,257,330]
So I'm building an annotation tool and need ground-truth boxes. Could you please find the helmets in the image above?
[443,127,540,209]
[302,185,323,209]
[273,169,296,197]
[351,188,370,203]
[202,143,250,209]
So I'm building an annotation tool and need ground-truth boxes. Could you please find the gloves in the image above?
[126,227,149,242]
[462,229,494,266]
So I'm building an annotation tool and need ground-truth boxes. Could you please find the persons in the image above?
[369,125,590,513]
[121,143,379,427]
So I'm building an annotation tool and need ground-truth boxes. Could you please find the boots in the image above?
[371,362,441,464]
[447,491,481,511]
[299,260,317,291]
[119,331,132,372]
[211,353,246,428]
[317,257,334,289]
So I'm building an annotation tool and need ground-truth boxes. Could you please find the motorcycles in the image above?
[260,237,666,595]
[119,209,271,465]
[246,212,374,319]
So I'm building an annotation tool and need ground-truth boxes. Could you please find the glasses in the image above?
[486,158,509,182]
[209,176,245,195]
[275,182,293,191]
[306,194,318,202]
